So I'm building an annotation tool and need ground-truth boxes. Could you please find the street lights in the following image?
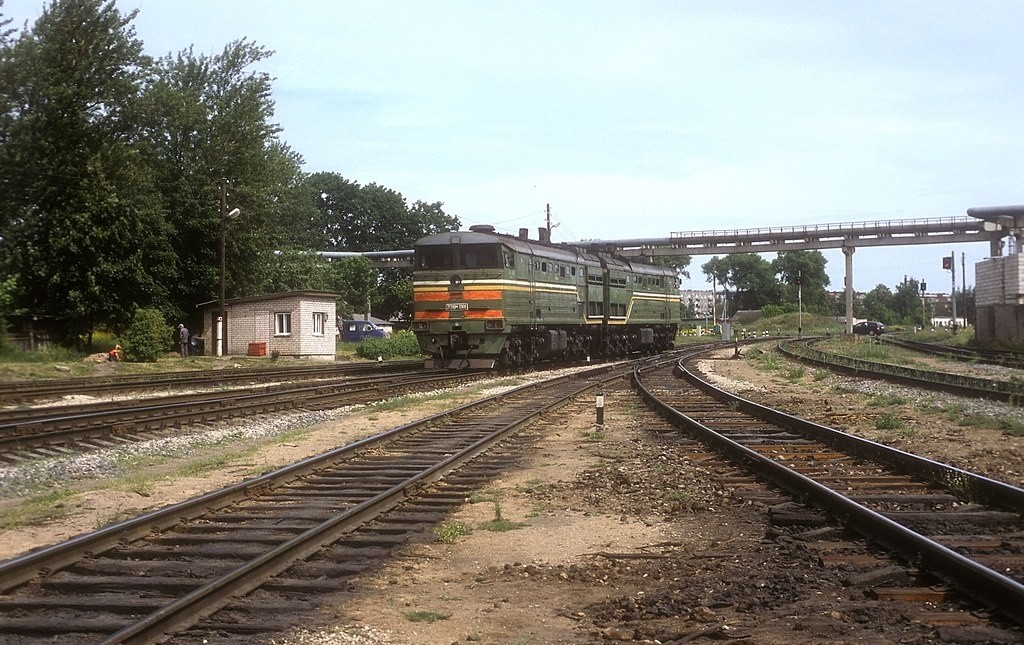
[220,207,240,352]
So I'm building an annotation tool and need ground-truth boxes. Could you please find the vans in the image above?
[342,321,391,342]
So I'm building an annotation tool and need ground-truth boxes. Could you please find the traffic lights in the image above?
[797,277,801,285]
[921,283,926,290]
[943,257,952,269]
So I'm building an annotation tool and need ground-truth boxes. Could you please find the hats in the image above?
[178,324,184,328]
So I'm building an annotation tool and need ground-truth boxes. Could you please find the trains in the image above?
[412,223,683,372]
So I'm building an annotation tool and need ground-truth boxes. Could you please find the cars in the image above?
[845,321,885,337]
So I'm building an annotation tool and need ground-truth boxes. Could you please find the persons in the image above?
[177,324,190,358]
[191,333,204,355]
